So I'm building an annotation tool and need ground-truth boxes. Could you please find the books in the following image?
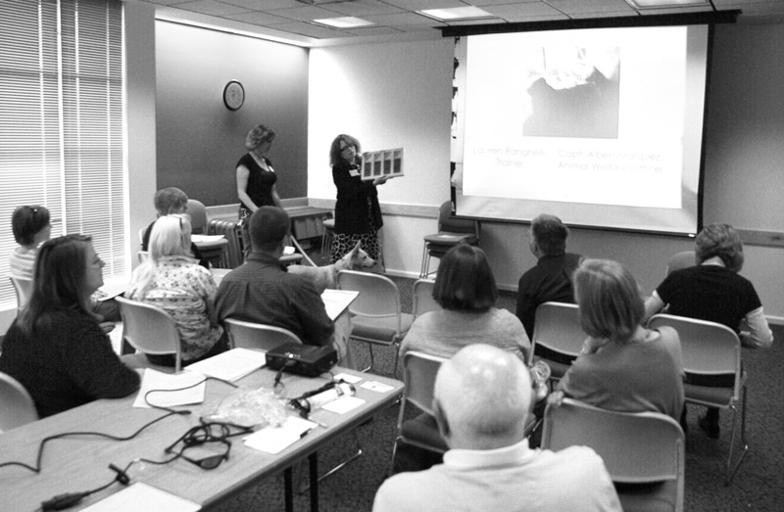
[320,288,362,323]
[92,275,128,303]
[105,321,126,357]
[77,480,201,512]
[191,234,226,244]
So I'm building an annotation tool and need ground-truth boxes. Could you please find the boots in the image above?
[697,406,721,438]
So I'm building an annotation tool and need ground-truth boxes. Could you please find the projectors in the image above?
[260,340,340,378]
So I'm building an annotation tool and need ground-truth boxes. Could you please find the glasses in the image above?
[340,143,353,153]
[166,434,231,470]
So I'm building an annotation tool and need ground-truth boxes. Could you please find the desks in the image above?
[0,347,407,512]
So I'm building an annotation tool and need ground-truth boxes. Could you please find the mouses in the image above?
[293,398,311,413]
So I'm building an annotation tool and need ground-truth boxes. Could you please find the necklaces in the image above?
[253,150,265,162]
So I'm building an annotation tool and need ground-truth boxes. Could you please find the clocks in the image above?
[223,80,245,111]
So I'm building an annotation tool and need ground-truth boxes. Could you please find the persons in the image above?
[397,244,537,431]
[547,258,685,496]
[0,234,141,418]
[330,134,389,271]
[517,213,593,365]
[235,124,284,262]
[124,214,229,368]
[640,223,775,438]
[214,205,374,428]
[370,342,626,512]
[141,188,209,267]
[9,204,136,354]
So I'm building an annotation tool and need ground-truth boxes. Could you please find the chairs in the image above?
[526,301,591,392]
[335,268,414,379]
[419,201,481,282]
[187,197,228,268]
[137,223,148,245]
[113,295,183,375]
[646,313,749,487]
[5,272,38,314]
[279,241,303,265]
[132,249,148,268]
[0,368,38,433]
[390,351,544,475]
[221,314,362,495]
[407,279,438,325]
[539,399,686,511]
[321,218,336,261]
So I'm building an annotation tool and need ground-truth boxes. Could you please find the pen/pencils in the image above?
[306,415,328,427]
